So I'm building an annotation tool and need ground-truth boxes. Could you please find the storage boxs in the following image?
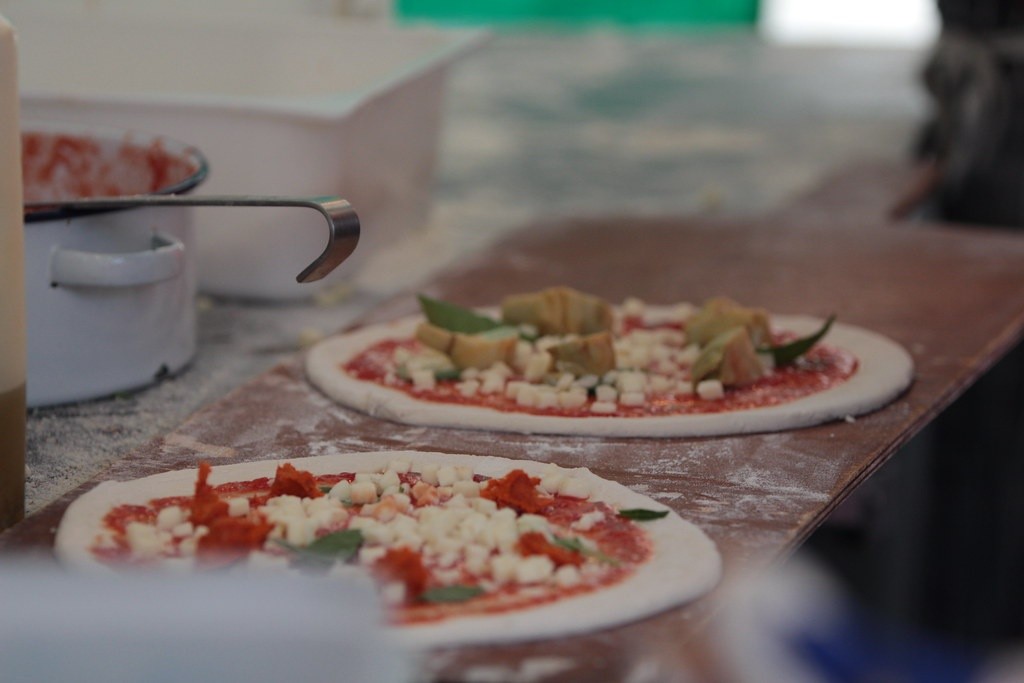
[15,16,499,303]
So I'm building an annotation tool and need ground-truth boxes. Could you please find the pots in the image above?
[20,130,210,404]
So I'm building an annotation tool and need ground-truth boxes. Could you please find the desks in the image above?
[16,30,941,642]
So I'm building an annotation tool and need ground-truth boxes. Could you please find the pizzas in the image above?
[304,285,915,437]
[54,450,722,649]
